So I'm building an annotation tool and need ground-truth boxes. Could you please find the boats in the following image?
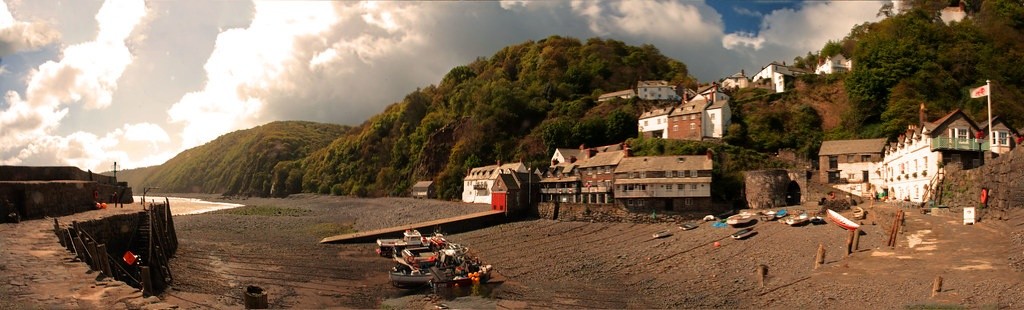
[852,205,865,219]
[826,208,860,231]
[679,222,699,230]
[766,210,777,221]
[776,208,789,219]
[376,226,493,289]
[810,215,824,225]
[652,230,673,239]
[726,212,757,225]
[731,226,758,240]
[786,211,810,226]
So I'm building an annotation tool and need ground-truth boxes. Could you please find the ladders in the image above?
[137,203,173,282]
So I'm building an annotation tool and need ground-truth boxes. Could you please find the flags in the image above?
[970,84,989,98]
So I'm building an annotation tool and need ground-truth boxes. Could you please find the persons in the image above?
[871,191,888,204]
[1003,127,1024,148]
[974,129,984,139]
[112,192,119,208]
